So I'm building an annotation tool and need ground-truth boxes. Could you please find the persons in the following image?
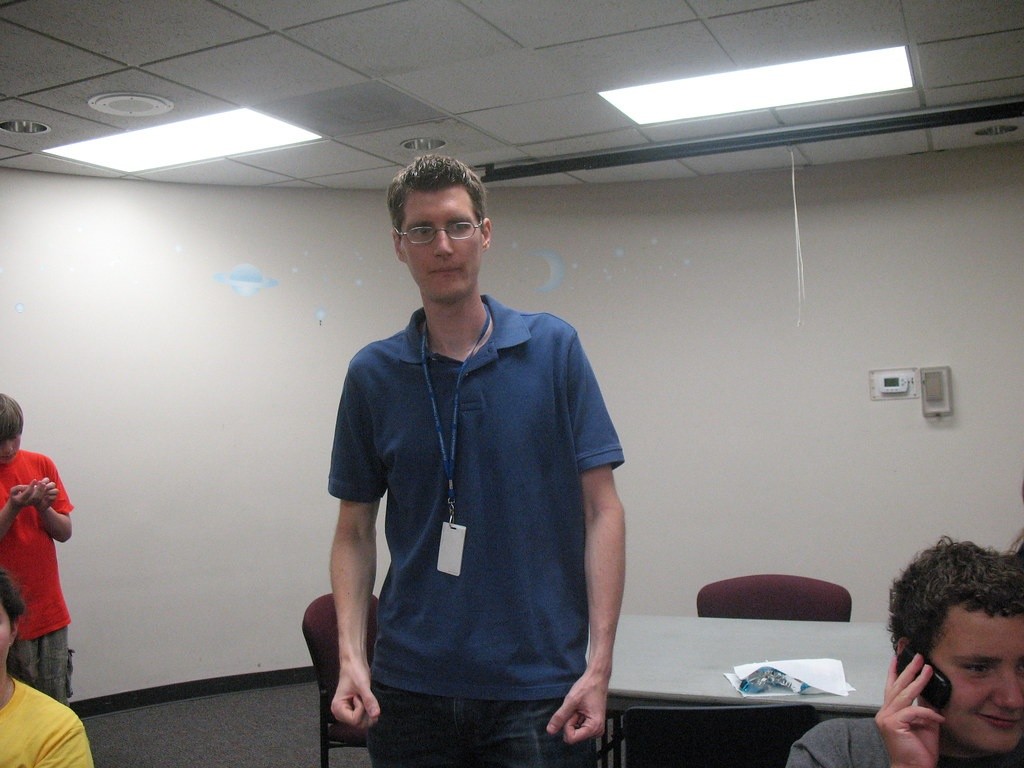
[329,153,626,768]
[0,393,75,768]
[0,571,95,768]
[784,529,1024,768]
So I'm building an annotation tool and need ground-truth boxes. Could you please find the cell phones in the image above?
[896,644,953,710]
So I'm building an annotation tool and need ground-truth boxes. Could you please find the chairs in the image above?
[623,704,817,767]
[302,592,379,768]
[696,574,853,623]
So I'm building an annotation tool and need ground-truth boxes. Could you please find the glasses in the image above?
[392,217,482,244]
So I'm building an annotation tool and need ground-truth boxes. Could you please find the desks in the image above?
[585,614,896,768]
